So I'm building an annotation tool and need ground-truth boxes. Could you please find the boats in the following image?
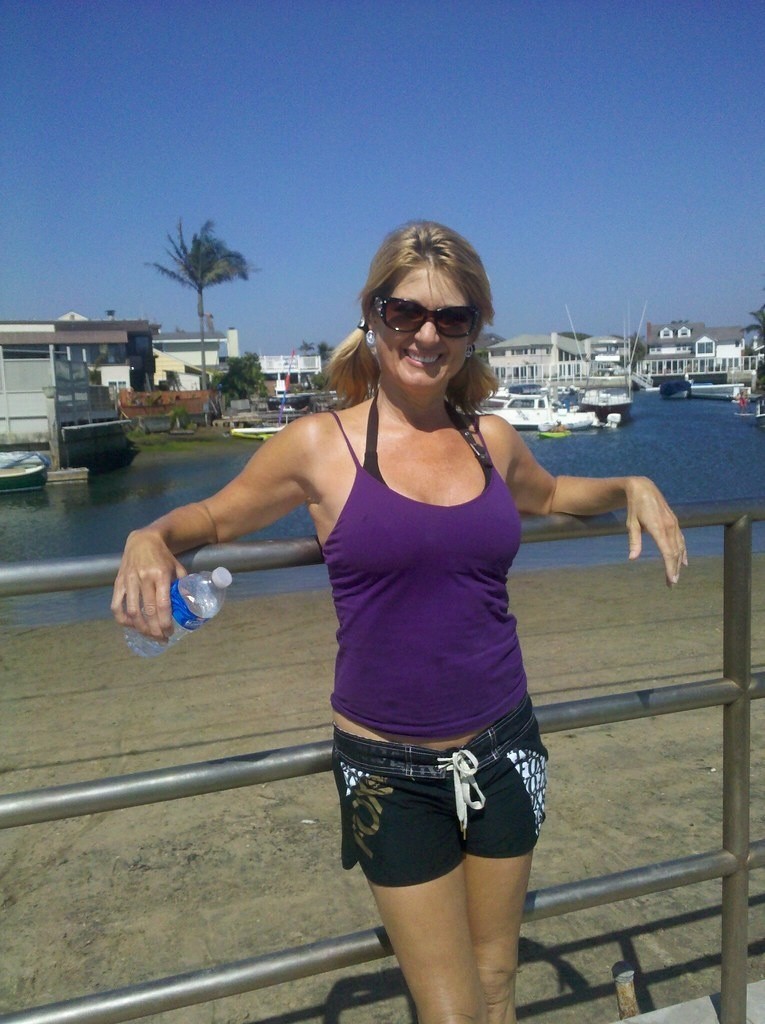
[472,393,599,432]
[230,425,288,442]
[657,381,752,402]
[1,456,49,493]
[564,296,648,419]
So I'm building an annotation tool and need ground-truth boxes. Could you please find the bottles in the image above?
[123,566,234,656]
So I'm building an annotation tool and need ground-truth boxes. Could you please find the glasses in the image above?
[372,295,480,339]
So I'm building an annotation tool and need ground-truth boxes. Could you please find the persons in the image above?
[111,219,688,1024]
